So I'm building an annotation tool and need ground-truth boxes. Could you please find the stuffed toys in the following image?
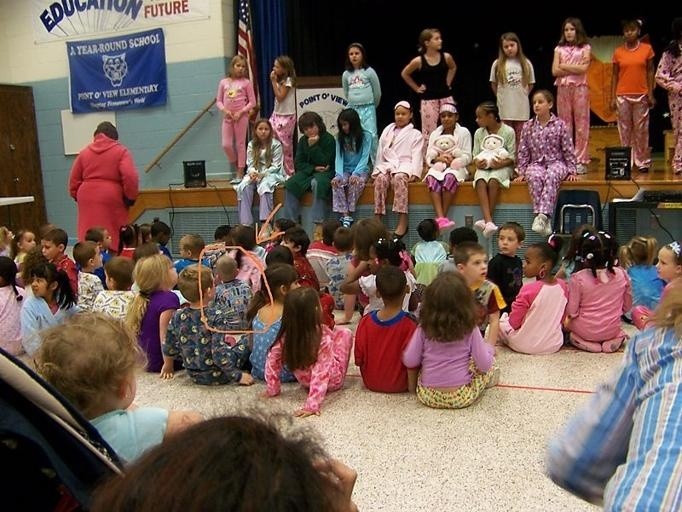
[474,134,509,169]
[430,135,468,170]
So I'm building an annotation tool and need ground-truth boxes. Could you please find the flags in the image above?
[236,0,262,125]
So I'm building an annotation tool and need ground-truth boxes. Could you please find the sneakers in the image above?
[601,336,626,353]
[482,222,499,239]
[672,165,682,174]
[474,219,485,232]
[341,216,355,229]
[569,332,602,353]
[258,222,272,240]
[575,163,588,175]
[532,212,548,234]
[336,215,344,228]
[312,222,324,242]
[435,217,456,237]
[541,219,553,238]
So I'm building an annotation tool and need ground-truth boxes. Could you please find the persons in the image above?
[517,89,576,237]
[217,53,257,183]
[472,103,516,237]
[268,55,298,177]
[609,18,655,173]
[341,43,382,168]
[370,100,424,238]
[233,118,288,226]
[401,29,455,170]
[489,32,536,144]
[421,102,472,232]
[277,111,336,224]
[71,120,139,252]
[1,216,680,419]
[90,407,357,512]
[331,108,374,228]
[551,17,592,175]
[39,309,201,463]
[655,36,682,175]
[539,274,680,511]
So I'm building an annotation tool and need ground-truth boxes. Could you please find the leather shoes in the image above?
[391,225,408,240]
[230,177,243,185]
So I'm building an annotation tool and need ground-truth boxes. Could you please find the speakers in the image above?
[183,160,206,187]
[605,146,632,180]
